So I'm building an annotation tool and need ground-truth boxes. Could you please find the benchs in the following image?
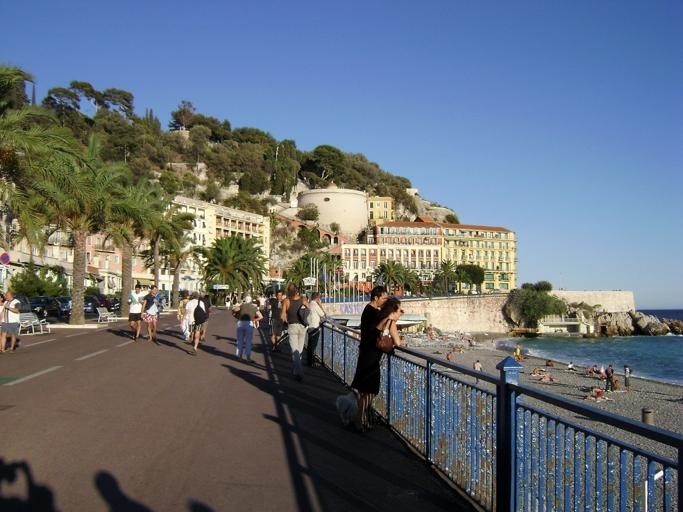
[96,307,117,323]
[18,312,50,336]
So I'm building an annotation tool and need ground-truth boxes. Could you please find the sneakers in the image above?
[336,394,355,427]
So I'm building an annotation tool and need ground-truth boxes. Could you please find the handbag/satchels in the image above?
[376,319,395,356]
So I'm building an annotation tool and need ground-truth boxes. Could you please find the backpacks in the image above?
[297,304,312,328]
[193,300,208,326]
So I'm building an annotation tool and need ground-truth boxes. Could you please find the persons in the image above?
[0,291,22,355]
[447,351,452,361]
[513,340,634,402]
[335,286,406,431]
[127,282,325,380]
[472,359,483,384]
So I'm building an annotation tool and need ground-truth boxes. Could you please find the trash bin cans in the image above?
[400,336,405,344]
[546,360,552,366]
[642,408,654,425]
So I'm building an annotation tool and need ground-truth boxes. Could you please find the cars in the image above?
[15,296,121,322]
[490,288,501,294]
[468,290,477,295]
[160,290,173,307]
